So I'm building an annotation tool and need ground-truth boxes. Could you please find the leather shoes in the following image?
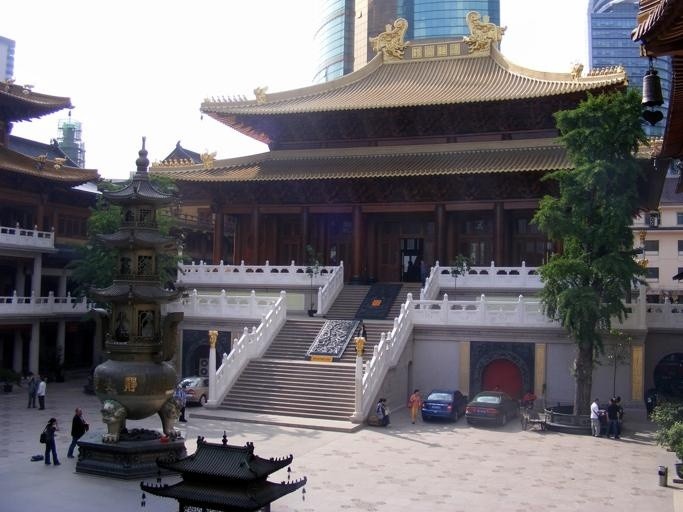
[68,455,74,458]
[27,406,45,410]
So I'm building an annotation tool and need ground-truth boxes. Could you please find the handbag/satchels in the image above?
[40,426,47,443]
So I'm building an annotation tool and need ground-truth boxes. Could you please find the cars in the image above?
[465,391,519,427]
[178,376,209,406]
[421,389,468,423]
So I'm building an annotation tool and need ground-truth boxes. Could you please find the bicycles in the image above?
[520,407,532,430]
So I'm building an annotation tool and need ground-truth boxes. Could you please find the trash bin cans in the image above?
[658,466,667,487]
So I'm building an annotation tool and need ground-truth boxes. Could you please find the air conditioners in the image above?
[199,358,209,377]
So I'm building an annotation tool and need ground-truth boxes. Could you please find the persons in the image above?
[407,261,417,282]
[44,417,62,465]
[177,385,196,422]
[27,375,49,410]
[492,384,502,392]
[375,398,392,428]
[590,395,625,440]
[356,324,367,342]
[409,389,421,424]
[67,407,86,458]
